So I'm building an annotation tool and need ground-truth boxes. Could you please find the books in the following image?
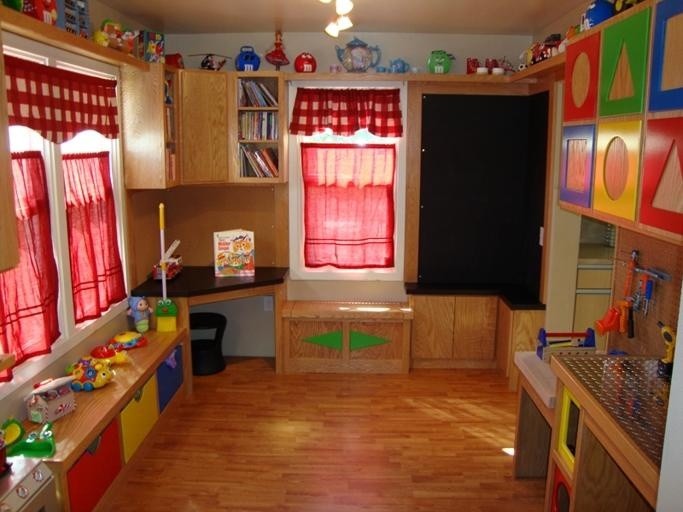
[164,107,176,186]
[236,78,278,178]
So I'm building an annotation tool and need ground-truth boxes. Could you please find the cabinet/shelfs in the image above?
[498,296,544,379]
[179,69,231,186]
[122,62,179,191]
[232,72,288,184]
[411,296,498,368]
[572,264,612,352]
[557,1,682,247]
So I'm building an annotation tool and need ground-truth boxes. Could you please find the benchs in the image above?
[0,326,184,511]
[282,300,409,375]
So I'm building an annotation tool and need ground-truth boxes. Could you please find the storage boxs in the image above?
[67,417,123,512]
[120,373,157,463]
[156,343,184,412]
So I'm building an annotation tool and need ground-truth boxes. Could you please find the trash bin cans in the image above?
[188,312,228,376]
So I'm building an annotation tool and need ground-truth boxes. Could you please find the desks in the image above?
[510,347,673,512]
[128,263,285,395]
